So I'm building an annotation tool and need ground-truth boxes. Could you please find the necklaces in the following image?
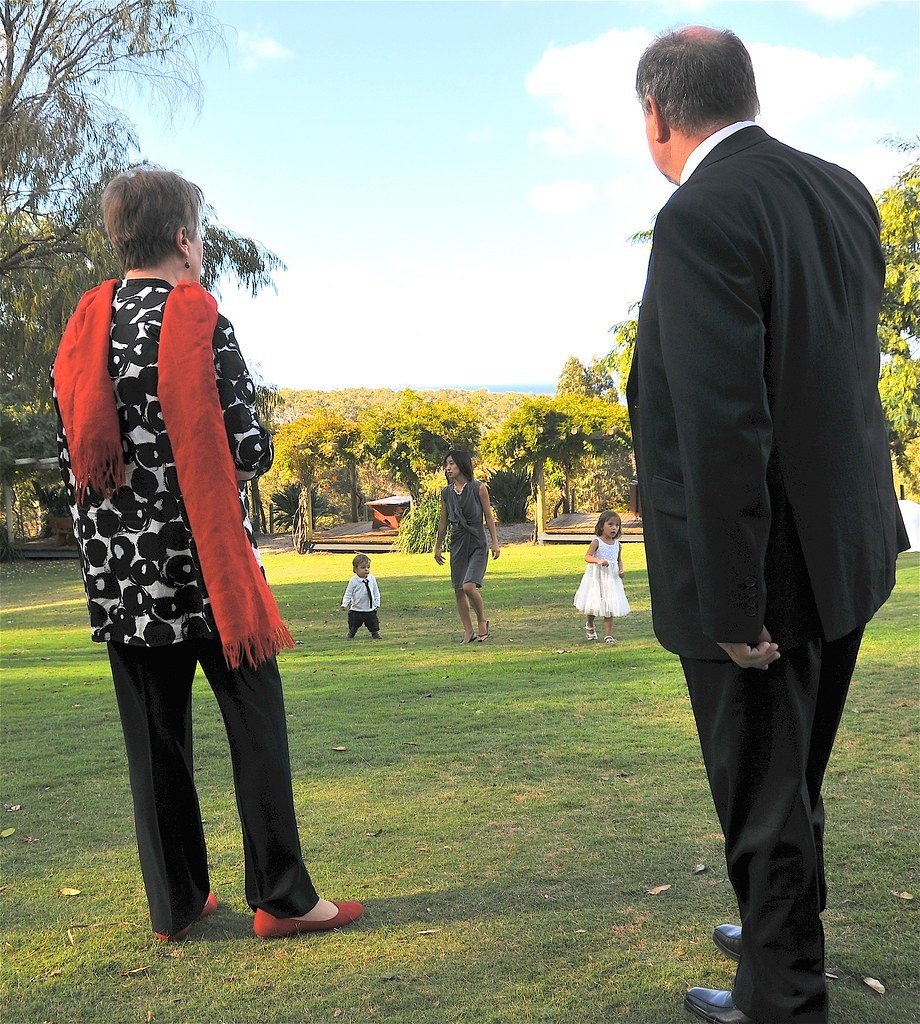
[452,479,469,496]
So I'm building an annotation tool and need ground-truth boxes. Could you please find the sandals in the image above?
[585,621,598,640]
[604,635,617,644]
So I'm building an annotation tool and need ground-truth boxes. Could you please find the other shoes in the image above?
[254,901,365,939]
[459,632,477,646]
[158,891,218,942]
[479,622,490,642]
[346,630,357,639]
[371,631,382,639]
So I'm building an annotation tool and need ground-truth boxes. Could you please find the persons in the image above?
[432,450,500,647]
[622,26,913,1024]
[572,509,631,644]
[340,554,383,641]
[45,166,367,942]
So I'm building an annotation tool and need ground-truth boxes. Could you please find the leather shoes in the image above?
[713,923,743,962]
[685,986,755,1024]
